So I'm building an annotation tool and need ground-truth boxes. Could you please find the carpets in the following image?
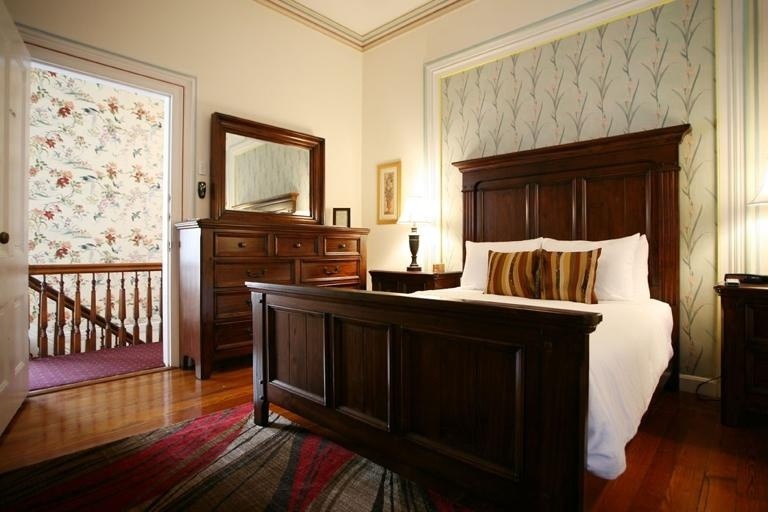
[27,341,170,391]
[0,399,480,512]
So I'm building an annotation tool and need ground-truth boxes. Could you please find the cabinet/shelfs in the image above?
[174,218,370,380]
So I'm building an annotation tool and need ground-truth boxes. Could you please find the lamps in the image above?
[395,196,437,272]
[748,178,768,206]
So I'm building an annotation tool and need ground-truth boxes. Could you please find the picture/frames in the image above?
[375,160,402,225]
[333,207,349,227]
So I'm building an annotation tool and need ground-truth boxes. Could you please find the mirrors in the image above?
[210,112,326,224]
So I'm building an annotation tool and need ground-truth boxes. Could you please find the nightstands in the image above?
[369,269,463,293]
[712,273,768,428]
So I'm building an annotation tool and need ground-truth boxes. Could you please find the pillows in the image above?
[538,246,602,305]
[640,234,650,301]
[459,236,541,291]
[542,233,640,302]
[483,250,536,298]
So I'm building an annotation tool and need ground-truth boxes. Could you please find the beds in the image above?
[243,123,691,512]
